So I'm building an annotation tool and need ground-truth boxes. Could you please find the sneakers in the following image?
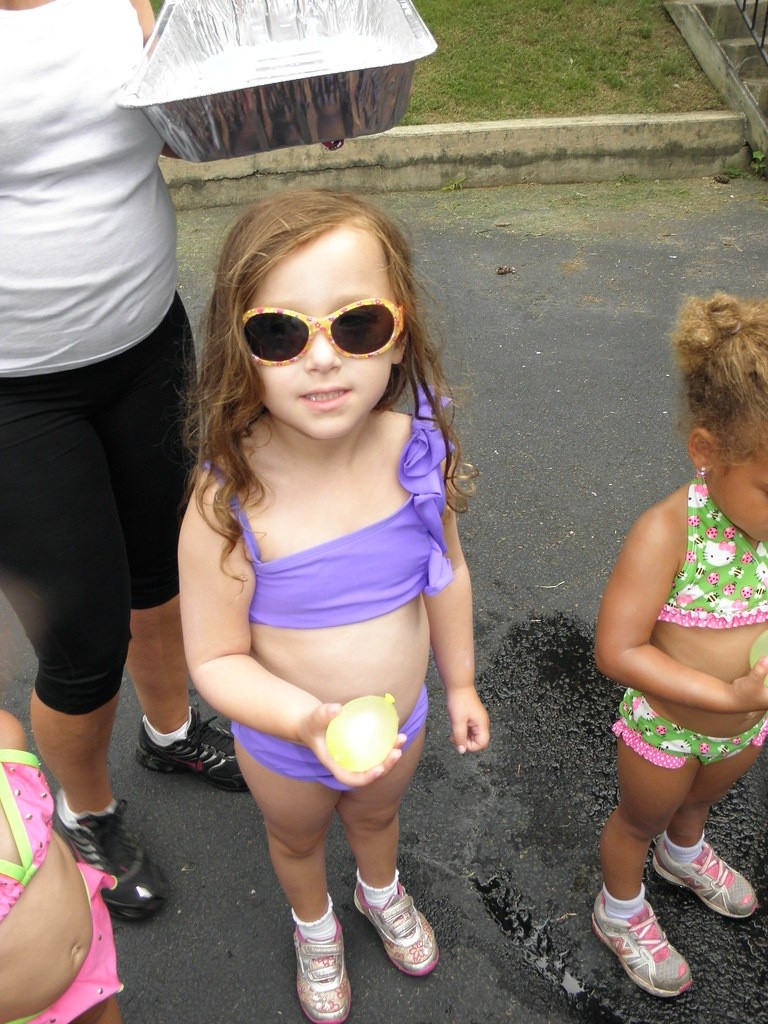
[653,833,757,917]
[136,704,252,792]
[352,877,440,977]
[591,890,693,995]
[292,914,351,1024]
[53,798,173,923]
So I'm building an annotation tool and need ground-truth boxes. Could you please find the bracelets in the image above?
[321,141,343,150]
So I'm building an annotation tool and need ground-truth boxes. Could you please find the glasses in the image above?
[239,299,404,366]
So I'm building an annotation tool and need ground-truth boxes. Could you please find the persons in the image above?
[179,191,489,1024]
[593,290,767,997]
[0,0,343,924]
[0,710,127,1023]
